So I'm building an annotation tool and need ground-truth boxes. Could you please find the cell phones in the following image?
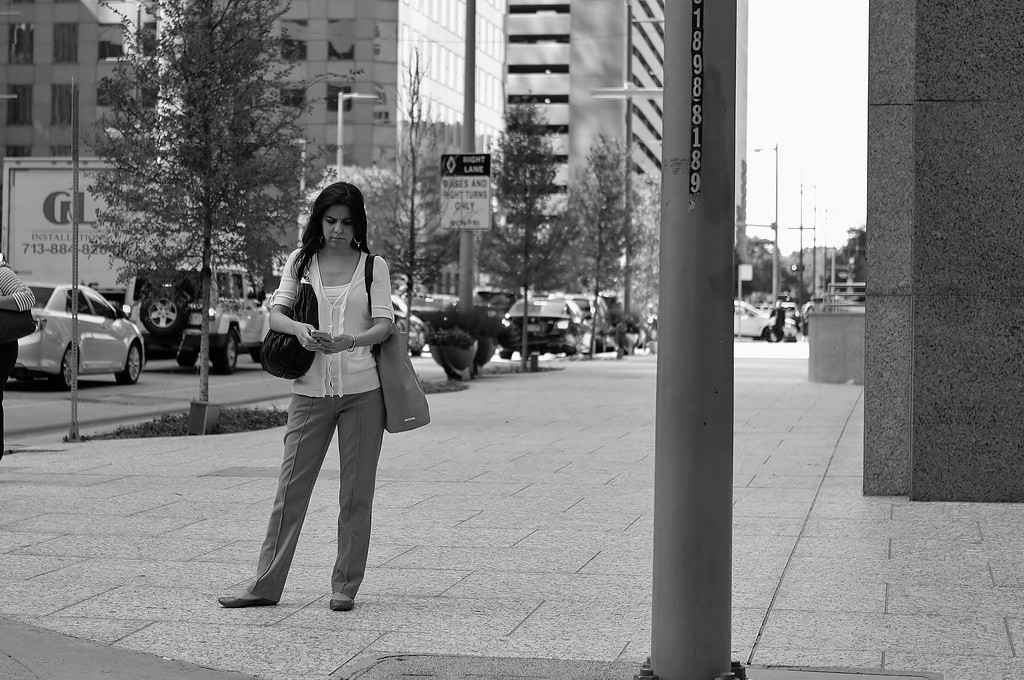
[310,330,331,343]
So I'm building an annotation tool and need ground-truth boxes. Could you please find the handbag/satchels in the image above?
[0,308,37,344]
[770,309,778,327]
[259,242,319,380]
[364,253,430,433]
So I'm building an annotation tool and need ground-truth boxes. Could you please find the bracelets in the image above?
[347,334,356,353]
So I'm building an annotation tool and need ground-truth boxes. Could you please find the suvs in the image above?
[123,262,271,375]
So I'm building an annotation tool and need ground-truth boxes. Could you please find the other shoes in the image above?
[329,591,355,611]
[218,596,278,608]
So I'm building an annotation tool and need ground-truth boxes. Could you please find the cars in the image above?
[733,299,798,342]
[389,288,647,361]
[9,280,146,391]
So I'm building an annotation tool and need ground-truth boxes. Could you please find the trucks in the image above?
[1,155,204,294]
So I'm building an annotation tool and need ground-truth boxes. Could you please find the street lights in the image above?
[753,142,780,309]
[334,92,380,184]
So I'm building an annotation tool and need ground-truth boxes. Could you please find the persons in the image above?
[769,300,786,342]
[0,259,36,461]
[217,182,393,611]
[89,283,100,293]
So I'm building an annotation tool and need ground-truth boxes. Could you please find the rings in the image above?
[302,344,306,347]
